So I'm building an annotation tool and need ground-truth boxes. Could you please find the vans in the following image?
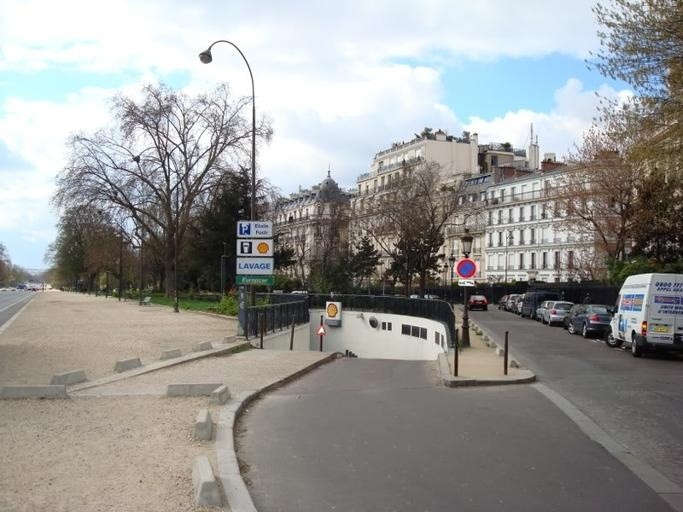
[605,273,683,356]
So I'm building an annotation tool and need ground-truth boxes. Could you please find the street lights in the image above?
[448,249,455,308]
[459,227,474,347]
[198,40,257,335]
[97,206,123,300]
[133,146,179,312]
[112,184,144,305]
[504,229,513,284]
[442,262,448,301]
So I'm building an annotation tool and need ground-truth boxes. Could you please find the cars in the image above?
[0,282,71,291]
[380,292,441,300]
[498,291,614,339]
[468,294,487,311]
